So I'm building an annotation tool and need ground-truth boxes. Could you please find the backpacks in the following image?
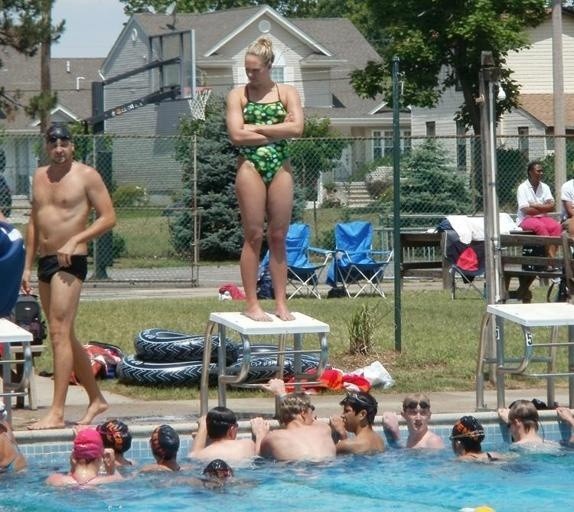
[10,294,47,357]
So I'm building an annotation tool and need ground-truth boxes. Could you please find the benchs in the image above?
[496,232,573,304]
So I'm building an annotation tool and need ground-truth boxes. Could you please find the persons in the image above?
[187,407,255,469]
[159,459,258,492]
[226,35,305,322]
[498,399,549,445]
[329,392,385,453]
[137,425,193,472]
[561,179,574,235]
[448,416,502,463]
[517,161,560,269]
[0,147,12,224]
[383,393,445,449]
[251,378,336,463]
[0,402,29,474]
[557,407,574,448]
[45,429,125,488]
[95,418,136,466]
[20,126,117,430]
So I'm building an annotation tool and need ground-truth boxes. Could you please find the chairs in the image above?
[285,223,330,300]
[326,221,394,300]
[441,225,489,300]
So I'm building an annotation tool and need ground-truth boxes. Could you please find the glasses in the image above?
[347,391,374,407]
[404,401,430,410]
[309,405,315,411]
[46,133,71,142]
[96,425,112,435]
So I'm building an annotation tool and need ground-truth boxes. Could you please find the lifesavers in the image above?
[226,343,332,383]
[116,354,218,386]
[134,328,238,366]
